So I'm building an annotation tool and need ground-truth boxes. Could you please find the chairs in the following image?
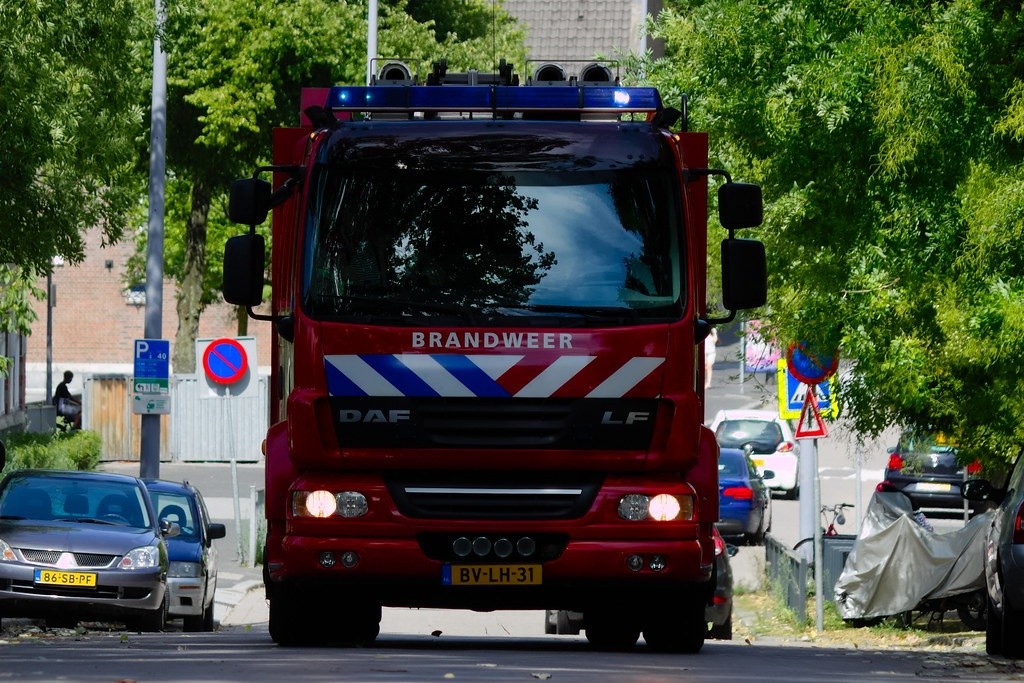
[97,495,136,525]
[14,488,56,518]
[158,505,193,531]
[63,494,89,518]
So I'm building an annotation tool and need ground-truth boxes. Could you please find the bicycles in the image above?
[792,503,855,565]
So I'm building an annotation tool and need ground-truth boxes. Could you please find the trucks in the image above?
[221,56,769,657]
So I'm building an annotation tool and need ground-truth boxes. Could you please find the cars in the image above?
[714,447,776,546]
[0,468,171,632]
[708,409,802,501]
[883,431,989,508]
[133,477,226,632]
[960,446,1024,660]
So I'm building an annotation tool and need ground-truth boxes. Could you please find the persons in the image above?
[704,327,720,389]
[53,371,81,430]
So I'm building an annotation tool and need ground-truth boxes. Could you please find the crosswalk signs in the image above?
[786,367,833,411]
